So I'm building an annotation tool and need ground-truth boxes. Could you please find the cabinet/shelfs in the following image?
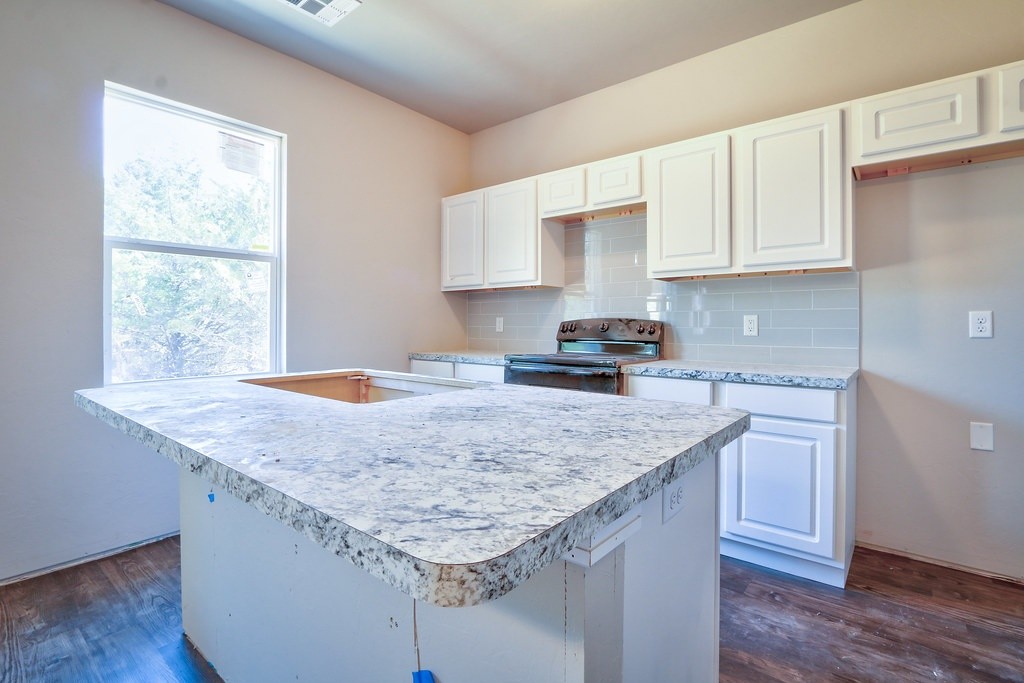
[851,62,1024,180]
[626,375,861,586]
[411,359,504,386]
[535,152,649,222]
[645,106,855,280]
[440,177,566,291]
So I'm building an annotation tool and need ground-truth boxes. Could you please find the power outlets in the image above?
[743,314,760,336]
[969,312,995,337]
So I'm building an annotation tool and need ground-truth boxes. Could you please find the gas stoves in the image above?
[503,318,664,368]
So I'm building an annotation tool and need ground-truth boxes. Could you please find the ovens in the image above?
[504,362,624,396]
